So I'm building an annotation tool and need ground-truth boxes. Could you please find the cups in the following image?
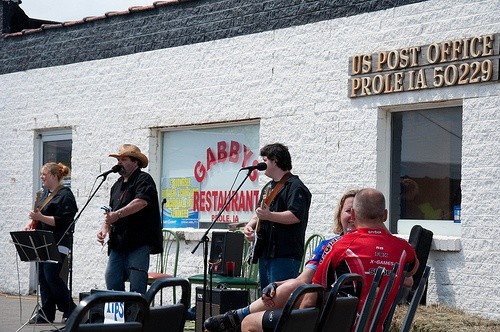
[226,261,235,277]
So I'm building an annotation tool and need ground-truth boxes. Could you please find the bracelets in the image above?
[116,209,124,219]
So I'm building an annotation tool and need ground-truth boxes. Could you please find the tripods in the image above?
[12,234,63,332]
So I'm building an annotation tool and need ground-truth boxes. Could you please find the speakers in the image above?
[208,231,244,276]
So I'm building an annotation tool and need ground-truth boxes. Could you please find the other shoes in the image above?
[29,316,53,324]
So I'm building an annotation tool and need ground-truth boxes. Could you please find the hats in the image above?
[109,144,148,168]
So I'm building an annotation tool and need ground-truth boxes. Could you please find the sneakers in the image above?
[204,310,241,332]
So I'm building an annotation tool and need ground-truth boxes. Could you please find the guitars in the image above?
[250,186,271,265]
[28,188,43,231]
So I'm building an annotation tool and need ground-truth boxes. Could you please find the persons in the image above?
[23,163,78,325]
[95,144,164,293]
[243,143,313,294]
[204,188,419,332]
[401,179,424,219]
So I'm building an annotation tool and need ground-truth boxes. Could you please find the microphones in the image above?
[242,162,267,171]
[98,165,121,178]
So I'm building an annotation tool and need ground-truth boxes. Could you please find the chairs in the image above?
[188,221,260,305]
[274,284,326,332]
[134,277,191,332]
[405,225,433,305]
[258,233,325,299]
[147,229,180,307]
[65,291,149,332]
[317,273,362,332]
